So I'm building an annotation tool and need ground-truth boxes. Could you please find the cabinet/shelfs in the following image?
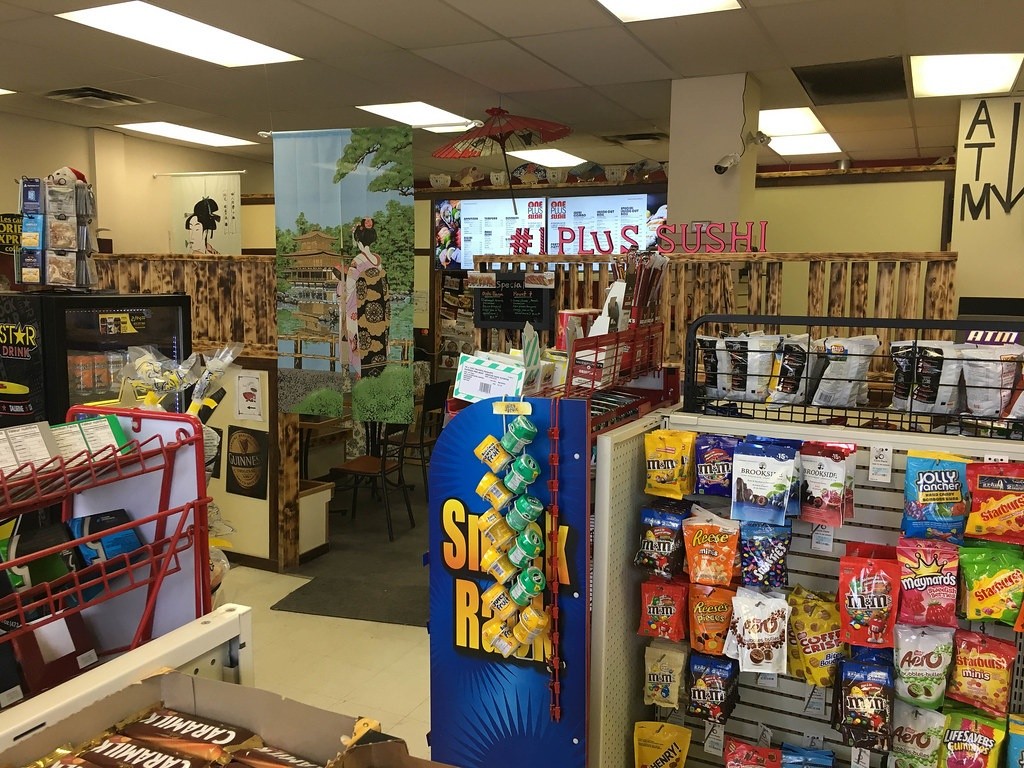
[0,405,213,713]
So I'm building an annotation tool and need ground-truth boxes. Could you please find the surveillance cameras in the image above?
[714,155,736,174]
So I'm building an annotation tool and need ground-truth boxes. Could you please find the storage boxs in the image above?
[452,321,624,403]
[0,667,463,768]
[0,421,62,510]
[50,414,132,469]
[0,509,146,638]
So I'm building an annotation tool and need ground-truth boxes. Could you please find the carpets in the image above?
[270,462,432,627]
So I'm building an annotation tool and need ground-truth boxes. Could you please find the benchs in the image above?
[299,425,352,565]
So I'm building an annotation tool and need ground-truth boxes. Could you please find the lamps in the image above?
[837,159,851,174]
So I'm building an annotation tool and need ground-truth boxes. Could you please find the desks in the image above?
[299,393,424,517]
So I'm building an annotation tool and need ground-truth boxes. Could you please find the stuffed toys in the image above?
[44,167,87,186]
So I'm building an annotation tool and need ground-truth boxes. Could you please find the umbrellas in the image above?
[432,108,574,215]
[611,253,668,377]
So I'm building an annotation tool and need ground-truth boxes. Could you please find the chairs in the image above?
[329,395,415,543]
[371,378,453,503]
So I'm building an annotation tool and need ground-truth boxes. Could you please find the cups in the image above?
[556,308,603,351]
